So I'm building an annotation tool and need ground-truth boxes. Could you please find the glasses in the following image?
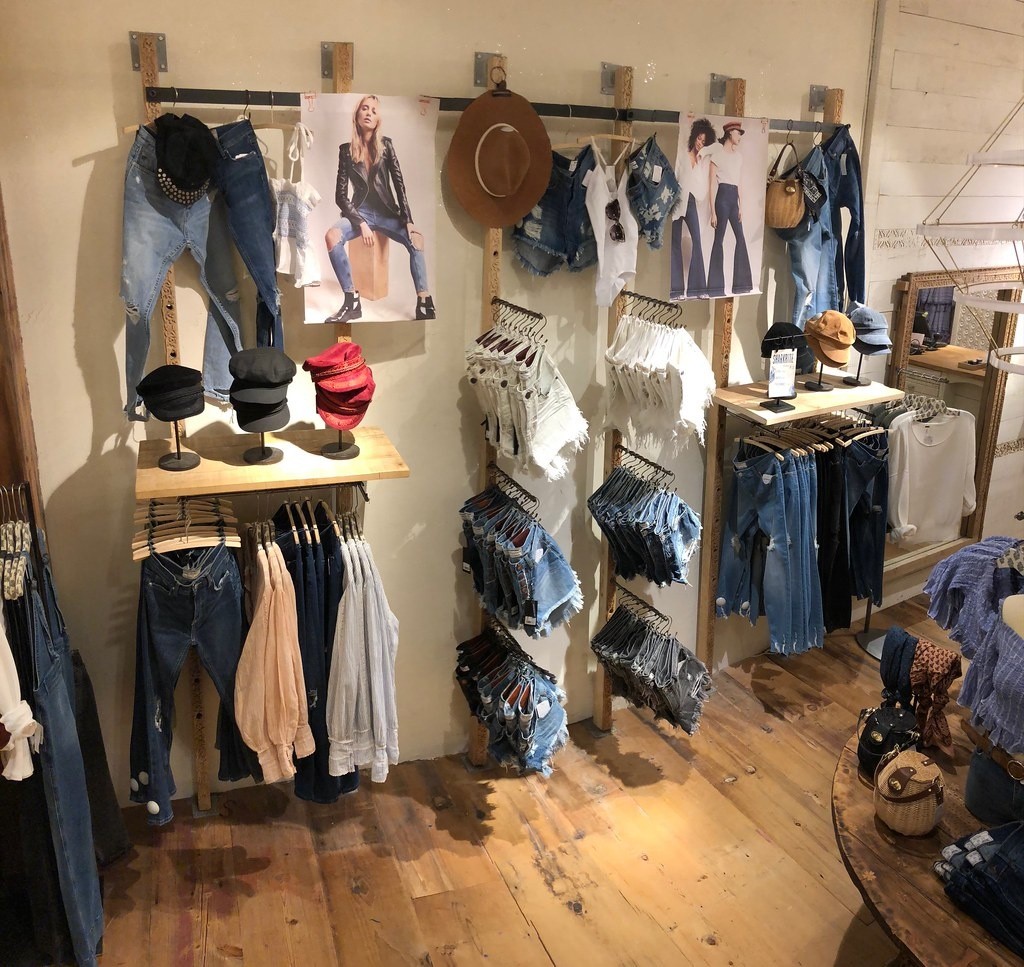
[606,199,626,242]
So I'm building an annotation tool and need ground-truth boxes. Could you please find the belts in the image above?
[960,718,1024,780]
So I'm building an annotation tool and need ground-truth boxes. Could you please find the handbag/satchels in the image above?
[774,170,831,244]
[765,143,805,228]
[857,706,920,778]
[872,744,948,836]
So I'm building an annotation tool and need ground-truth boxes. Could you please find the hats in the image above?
[302,343,370,392]
[847,307,893,345]
[229,348,296,404]
[805,310,856,363]
[136,365,205,408]
[315,367,376,431]
[723,122,745,135]
[804,330,848,368]
[149,397,204,422]
[761,322,812,369]
[852,337,892,355]
[449,90,553,229]
[155,113,217,206]
[230,396,290,432]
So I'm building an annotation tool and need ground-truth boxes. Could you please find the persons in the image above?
[670,113,753,299]
[325,94,437,322]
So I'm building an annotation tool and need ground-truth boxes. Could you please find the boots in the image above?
[416,295,437,319]
[325,291,362,322]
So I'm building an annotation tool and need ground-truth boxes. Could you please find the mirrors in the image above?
[883,266,1024,585]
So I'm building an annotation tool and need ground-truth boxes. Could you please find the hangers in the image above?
[485,617,532,671]
[618,290,684,328]
[0,482,28,601]
[615,588,673,637]
[489,465,546,519]
[772,120,823,173]
[737,397,960,461]
[548,105,643,162]
[131,500,370,564]
[477,295,550,363]
[158,83,186,117]
[611,446,679,493]
[237,91,310,134]
